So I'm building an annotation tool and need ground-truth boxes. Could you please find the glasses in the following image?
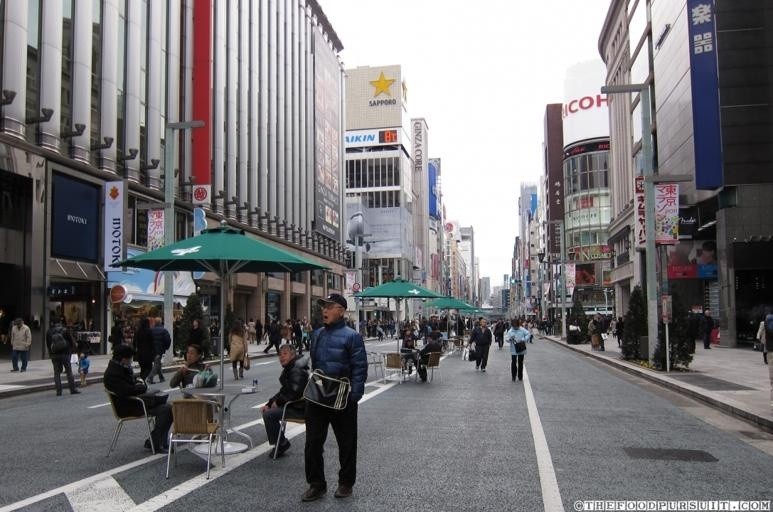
[321,305,344,310]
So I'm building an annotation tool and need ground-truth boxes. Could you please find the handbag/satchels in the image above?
[303,370,352,410]
[244,356,250,370]
[462,348,476,361]
[754,340,764,352]
[193,372,217,388]
[514,342,526,353]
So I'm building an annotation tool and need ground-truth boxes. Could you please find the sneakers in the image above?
[270,438,352,501]
[145,439,174,454]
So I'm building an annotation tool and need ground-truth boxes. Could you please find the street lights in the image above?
[597,81,658,368]
[161,117,206,362]
[353,234,374,333]
[542,220,567,339]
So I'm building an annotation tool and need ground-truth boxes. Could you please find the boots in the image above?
[234,369,238,380]
[239,368,243,378]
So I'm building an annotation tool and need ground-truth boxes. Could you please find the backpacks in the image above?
[51,328,67,354]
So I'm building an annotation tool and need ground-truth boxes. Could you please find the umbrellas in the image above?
[108,217,333,471]
[424,297,488,337]
[348,275,444,357]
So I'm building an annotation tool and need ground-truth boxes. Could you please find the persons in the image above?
[10,309,773,457]
[299,290,367,501]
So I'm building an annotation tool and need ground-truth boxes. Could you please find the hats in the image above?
[317,294,347,309]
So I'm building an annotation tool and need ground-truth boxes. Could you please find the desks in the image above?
[179,385,260,456]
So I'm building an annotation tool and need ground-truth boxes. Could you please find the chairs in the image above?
[164,399,225,480]
[270,396,307,460]
[102,384,158,454]
[365,332,470,388]
